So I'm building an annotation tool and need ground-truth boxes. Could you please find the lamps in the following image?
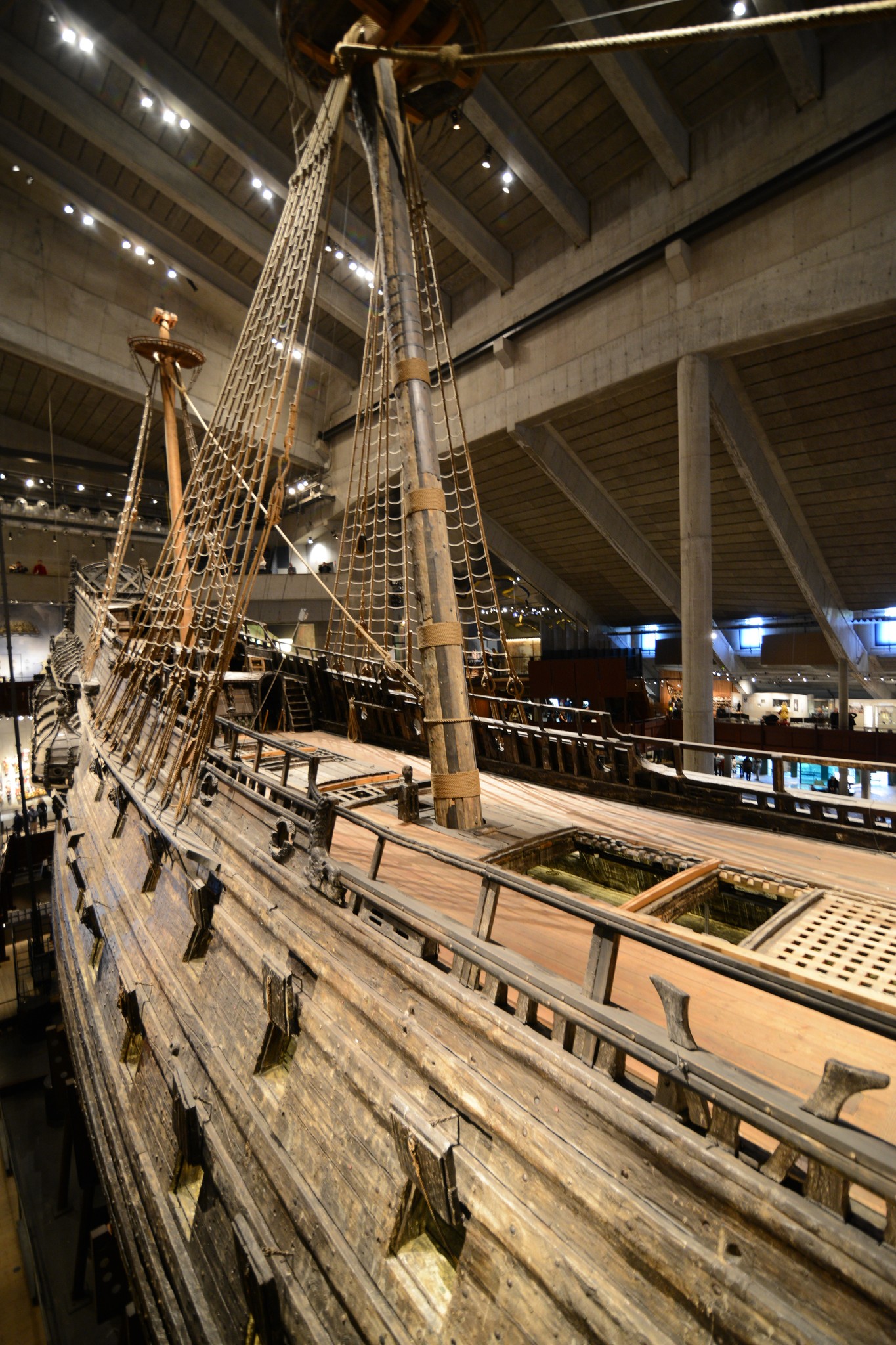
[131,544,135,551]
[91,540,95,547]
[480,148,494,171]
[8,532,13,540]
[308,537,313,544]
[53,535,57,544]
[449,112,462,130]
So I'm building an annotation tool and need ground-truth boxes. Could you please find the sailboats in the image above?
[31,12,895,1345]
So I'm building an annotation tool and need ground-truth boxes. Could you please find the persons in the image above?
[652,697,858,795]
[481,648,593,724]
[9,560,47,575]
[10,797,48,836]
[257,555,330,574]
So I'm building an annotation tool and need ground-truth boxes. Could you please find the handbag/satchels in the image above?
[746,759,753,767]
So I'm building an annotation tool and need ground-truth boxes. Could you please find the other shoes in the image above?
[755,778,759,781]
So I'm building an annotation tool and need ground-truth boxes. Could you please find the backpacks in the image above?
[754,757,762,763]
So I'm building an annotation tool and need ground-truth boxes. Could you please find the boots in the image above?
[746,775,749,781]
[749,775,751,781]
